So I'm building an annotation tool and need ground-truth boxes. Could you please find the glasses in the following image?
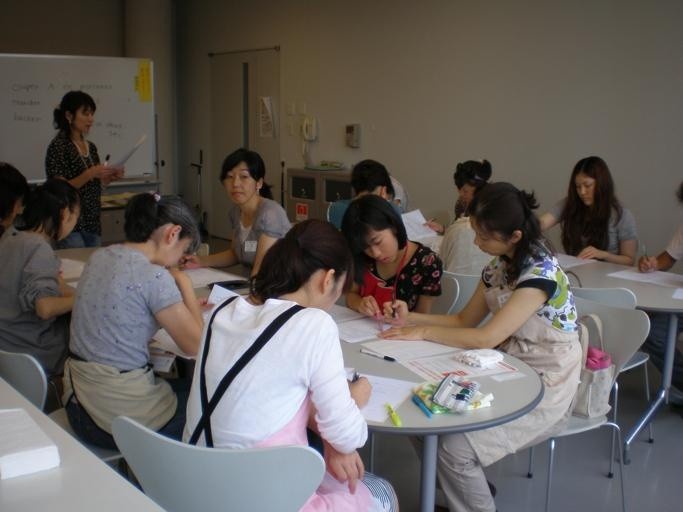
[456,163,486,182]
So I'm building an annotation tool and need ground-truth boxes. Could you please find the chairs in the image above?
[1,350,130,480]
[430,259,492,325]
[111,414,328,510]
[526,286,653,478]
[549,295,653,511]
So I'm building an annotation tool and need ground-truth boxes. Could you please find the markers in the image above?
[103,154,110,167]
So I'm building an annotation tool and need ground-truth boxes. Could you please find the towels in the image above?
[412,379,494,414]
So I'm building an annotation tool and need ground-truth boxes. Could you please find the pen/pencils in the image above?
[352,372,359,382]
[642,241,649,261]
[183,247,191,264]
[385,402,401,427]
[360,348,396,361]
[391,289,395,318]
[423,218,437,226]
[376,312,383,331]
[413,394,433,418]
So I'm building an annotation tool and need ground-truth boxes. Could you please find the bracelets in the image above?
[87,168,92,181]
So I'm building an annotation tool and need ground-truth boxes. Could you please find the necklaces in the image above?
[72,138,89,158]
[71,141,93,171]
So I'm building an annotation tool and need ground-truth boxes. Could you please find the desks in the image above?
[0,371,168,510]
[549,249,681,464]
[40,241,545,511]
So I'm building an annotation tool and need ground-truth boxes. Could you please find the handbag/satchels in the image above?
[572,314,616,419]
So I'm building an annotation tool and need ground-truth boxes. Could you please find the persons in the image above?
[435,182,498,276]
[536,154,639,267]
[337,193,444,325]
[177,146,292,282]
[638,181,681,417]
[374,180,585,511]
[326,158,395,232]
[422,157,493,236]
[0,179,83,379]
[43,90,126,249]
[0,162,29,241]
[175,217,400,511]
[60,189,206,450]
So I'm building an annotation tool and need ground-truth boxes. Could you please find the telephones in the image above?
[302,114,317,141]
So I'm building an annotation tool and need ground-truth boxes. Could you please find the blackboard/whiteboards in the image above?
[0,53,163,187]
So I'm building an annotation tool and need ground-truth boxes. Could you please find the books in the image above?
[108,134,147,175]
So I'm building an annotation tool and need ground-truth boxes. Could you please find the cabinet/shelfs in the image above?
[284,163,357,223]
